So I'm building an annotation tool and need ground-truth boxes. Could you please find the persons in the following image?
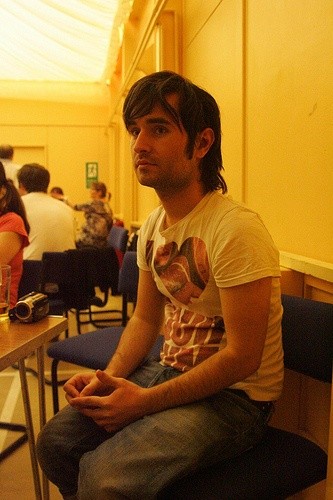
[0,145,114,316]
[35,71,285,500]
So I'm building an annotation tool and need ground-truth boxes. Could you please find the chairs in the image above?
[46,249,164,415]
[157,295,333,500]
[36,225,140,341]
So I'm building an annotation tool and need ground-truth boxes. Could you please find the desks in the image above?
[0,311,68,500]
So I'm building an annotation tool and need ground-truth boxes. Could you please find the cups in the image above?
[0,264,11,321]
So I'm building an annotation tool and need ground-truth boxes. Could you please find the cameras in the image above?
[13,292,49,323]
